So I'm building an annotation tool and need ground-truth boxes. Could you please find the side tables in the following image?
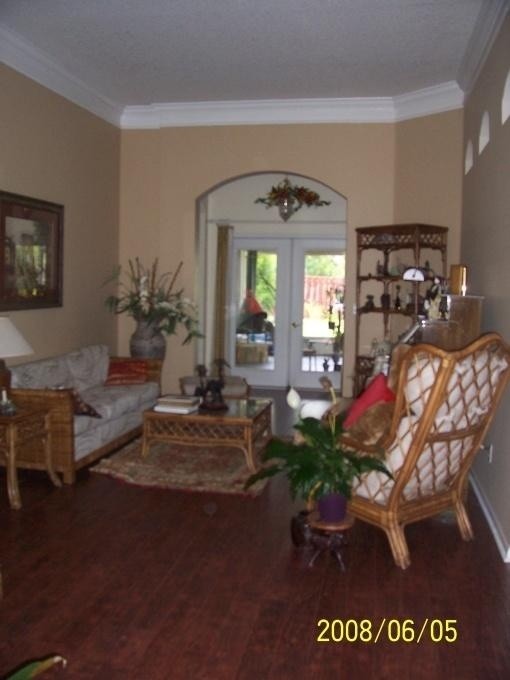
[0,398,62,511]
[306,511,354,576]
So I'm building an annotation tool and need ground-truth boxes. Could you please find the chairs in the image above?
[295,332,510,571]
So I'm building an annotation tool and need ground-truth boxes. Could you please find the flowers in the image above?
[102,257,205,345]
[243,387,396,502]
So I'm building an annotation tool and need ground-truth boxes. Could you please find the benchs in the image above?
[179,375,249,397]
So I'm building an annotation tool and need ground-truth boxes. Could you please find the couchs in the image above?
[9,344,161,484]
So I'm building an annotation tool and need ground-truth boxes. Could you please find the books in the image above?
[449,265,467,296]
[154,395,203,414]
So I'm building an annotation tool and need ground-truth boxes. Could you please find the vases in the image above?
[129,320,165,397]
[317,495,346,521]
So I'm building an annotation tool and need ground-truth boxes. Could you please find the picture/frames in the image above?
[0,189,64,312]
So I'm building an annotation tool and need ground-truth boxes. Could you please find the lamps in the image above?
[251,180,330,227]
[0,317,34,412]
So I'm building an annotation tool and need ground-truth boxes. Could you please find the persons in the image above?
[427,276,442,321]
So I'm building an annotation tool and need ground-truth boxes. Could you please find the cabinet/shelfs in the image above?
[358,274,444,358]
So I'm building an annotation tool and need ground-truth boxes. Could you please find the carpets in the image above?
[89,433,274,499]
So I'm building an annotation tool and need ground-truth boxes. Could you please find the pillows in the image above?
[343,374,396,430]
[103,357,145,385]
